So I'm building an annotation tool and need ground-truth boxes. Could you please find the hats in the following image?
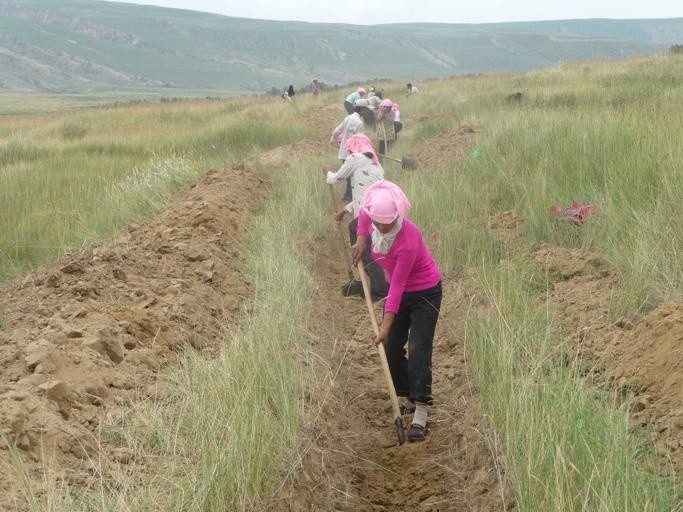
[356,99,375,108]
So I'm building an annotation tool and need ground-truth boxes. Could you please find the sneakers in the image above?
[399,403,416,415]
[409,422,429,440]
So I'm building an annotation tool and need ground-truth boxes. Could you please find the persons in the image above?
[404,83,420,99]
[324,133,390,298]
[349,179,443,443]
[329,99,375,202]
[343,85,402,156]
[311,79,319,96]
[288,85,294,97]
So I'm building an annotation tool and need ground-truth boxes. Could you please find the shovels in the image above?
[375,153,419,170]
[357,259,405,451]
[327,165,362,297]
